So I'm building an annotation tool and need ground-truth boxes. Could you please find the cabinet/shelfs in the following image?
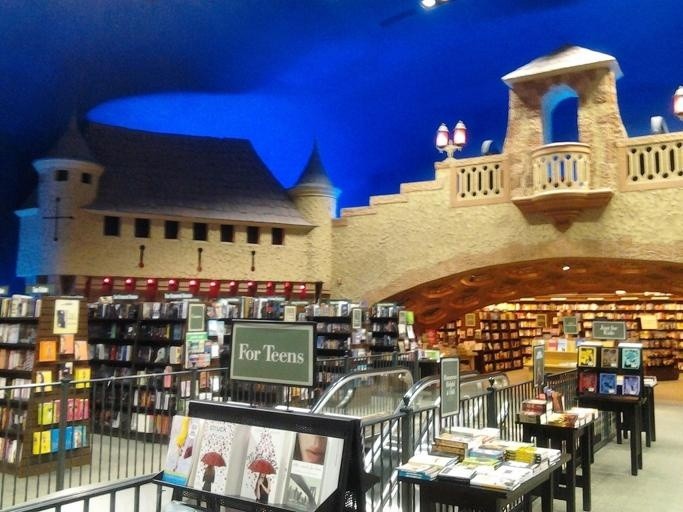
[90,296,205,444]
[210,300,423,409]
[472,300,683,381]
[1,293,94,477]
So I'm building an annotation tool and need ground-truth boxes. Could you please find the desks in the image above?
[395,387,656,512]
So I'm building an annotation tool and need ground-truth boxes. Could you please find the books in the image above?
[0,287,683,511]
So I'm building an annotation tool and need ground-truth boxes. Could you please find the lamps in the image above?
[436,121,468,162]
